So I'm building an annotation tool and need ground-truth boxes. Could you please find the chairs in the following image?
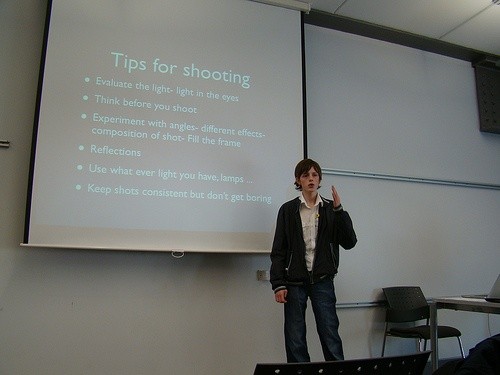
[381,286,465,360]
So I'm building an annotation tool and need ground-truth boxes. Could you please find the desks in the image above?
[430,296,500,372]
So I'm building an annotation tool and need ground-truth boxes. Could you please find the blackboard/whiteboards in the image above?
[314,172,500,308]
[300,22,500,191]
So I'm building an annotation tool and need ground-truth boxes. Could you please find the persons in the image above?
[270,159,358,364]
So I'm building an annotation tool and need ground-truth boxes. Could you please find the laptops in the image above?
[461,274,500,298]
